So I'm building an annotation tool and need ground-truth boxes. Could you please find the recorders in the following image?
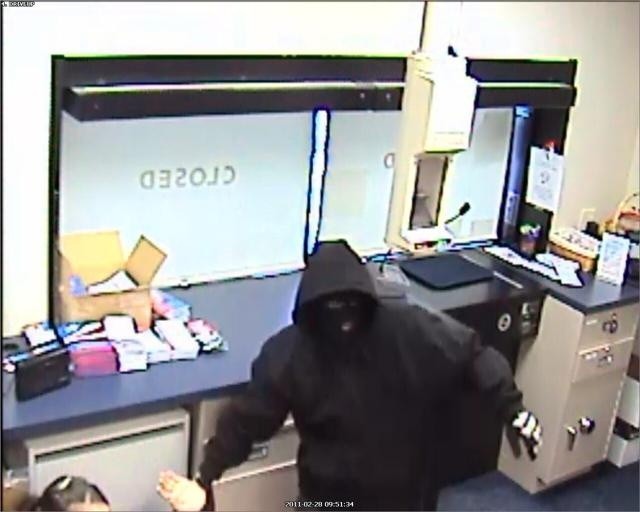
[11,338,69,402]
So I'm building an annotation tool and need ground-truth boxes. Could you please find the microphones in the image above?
[367,202,470,298]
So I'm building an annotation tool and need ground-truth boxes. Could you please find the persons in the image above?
[28,470,215,512]
[156,240,546,512]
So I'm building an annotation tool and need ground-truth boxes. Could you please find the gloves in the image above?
[503,407,544,462]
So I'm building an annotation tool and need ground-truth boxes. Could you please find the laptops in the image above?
[400,254,492,289]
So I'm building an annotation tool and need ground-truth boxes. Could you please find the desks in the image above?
[2,250,545,511]
